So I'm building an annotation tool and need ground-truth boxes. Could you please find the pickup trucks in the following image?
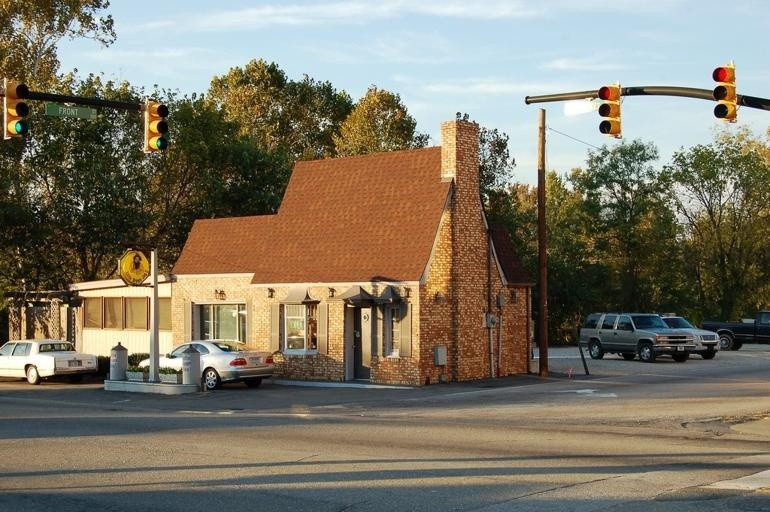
[700,310,770,351]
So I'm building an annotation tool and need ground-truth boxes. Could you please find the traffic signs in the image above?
[46,102,97,120]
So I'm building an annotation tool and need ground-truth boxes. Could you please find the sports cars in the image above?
[137,338,275,390]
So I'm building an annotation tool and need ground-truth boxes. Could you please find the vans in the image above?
[622,316,721,360]
[579,312,698,362]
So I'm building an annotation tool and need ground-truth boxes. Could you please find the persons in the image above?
[129,254,144,274]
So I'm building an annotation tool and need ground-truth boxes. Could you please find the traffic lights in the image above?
[597,84,622,139]
[711,65,737,124]
[144,98,169,153]
[4,80,29,139]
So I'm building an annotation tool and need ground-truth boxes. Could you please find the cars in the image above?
[0,339,98,384]
[287,334,304,349]
